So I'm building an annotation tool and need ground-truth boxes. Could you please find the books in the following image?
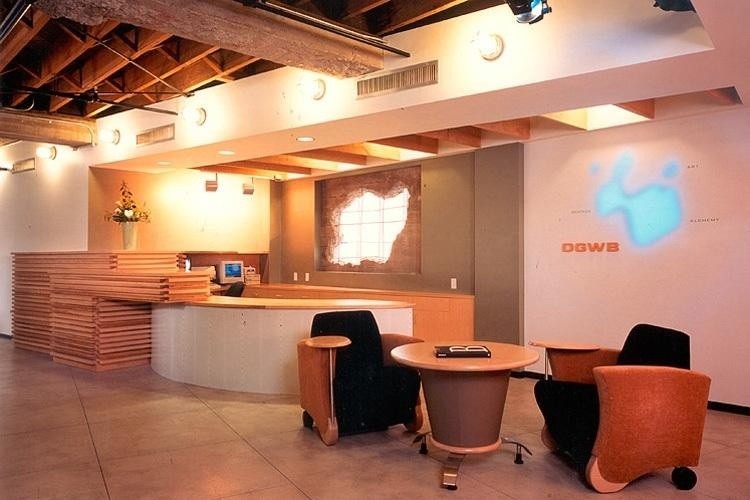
[433,344,491,359]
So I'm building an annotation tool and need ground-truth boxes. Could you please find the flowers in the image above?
[101,176,153,224]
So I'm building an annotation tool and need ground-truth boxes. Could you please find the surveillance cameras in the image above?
[505,0,552,25]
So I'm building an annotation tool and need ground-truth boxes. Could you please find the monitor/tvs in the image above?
[216,261,244,284]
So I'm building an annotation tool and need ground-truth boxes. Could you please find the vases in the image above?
[119,218,141,252]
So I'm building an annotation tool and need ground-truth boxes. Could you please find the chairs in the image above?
[296,308,424,448]
[529,323,714,496]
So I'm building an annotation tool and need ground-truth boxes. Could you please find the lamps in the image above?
[204,173,258,196]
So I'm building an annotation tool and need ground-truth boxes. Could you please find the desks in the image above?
[388,342,542,492]
